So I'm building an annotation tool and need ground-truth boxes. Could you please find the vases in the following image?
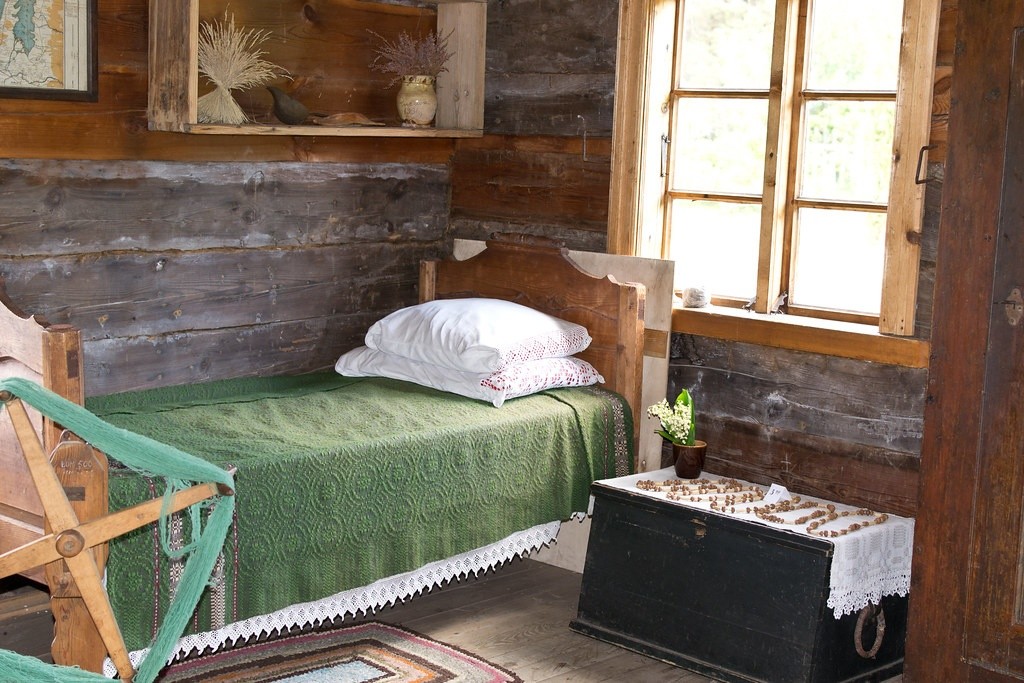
[672,440,707,479]
[396,75,438,128]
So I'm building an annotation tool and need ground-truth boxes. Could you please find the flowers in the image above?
[647,388,696,446]
[366,28,457,89]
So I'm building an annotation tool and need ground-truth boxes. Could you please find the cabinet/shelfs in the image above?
[569,466,915,683]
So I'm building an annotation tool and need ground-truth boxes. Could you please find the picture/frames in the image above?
[0,0,99,103]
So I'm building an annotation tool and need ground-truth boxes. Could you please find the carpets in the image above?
[154,620,524,683]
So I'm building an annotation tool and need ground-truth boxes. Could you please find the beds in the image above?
[0,231,648,674]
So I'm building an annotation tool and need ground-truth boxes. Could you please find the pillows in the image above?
[335,346,606,408]
[365,297,592,372]
